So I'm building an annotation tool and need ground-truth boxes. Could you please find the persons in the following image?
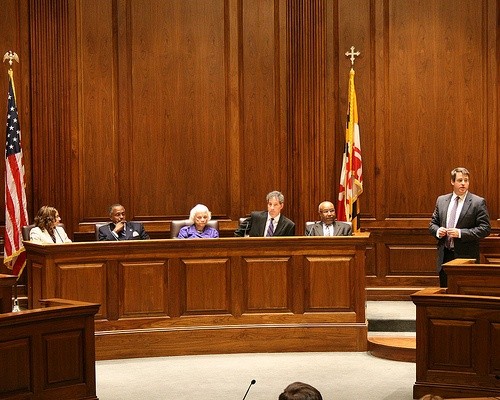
[30,205,72,244]
[428,168,491,288]
[278,382,323,400]
[305,201,352,236]
[232,191,295,237]
[178,204,219,239]
[99,204,151,241]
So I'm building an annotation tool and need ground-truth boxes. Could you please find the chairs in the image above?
[22,218,354,241]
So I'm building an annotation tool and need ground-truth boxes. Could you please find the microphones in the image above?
[107,226,118,241]
[243,380,255,400]
[244,219,251,237]
[53,226,63,243]
[187,227,190,239]
[308,221,317,236]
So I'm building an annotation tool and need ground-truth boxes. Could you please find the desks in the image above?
[411,235,500,400]
[0,297,101,400]
[22,232,370,361]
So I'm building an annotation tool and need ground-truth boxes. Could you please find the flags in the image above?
[3,69,31,282]
[337,70,364,234]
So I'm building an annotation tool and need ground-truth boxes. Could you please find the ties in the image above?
[325,224,330,236]
[446,196,461,250]
[266,218,275,236]
